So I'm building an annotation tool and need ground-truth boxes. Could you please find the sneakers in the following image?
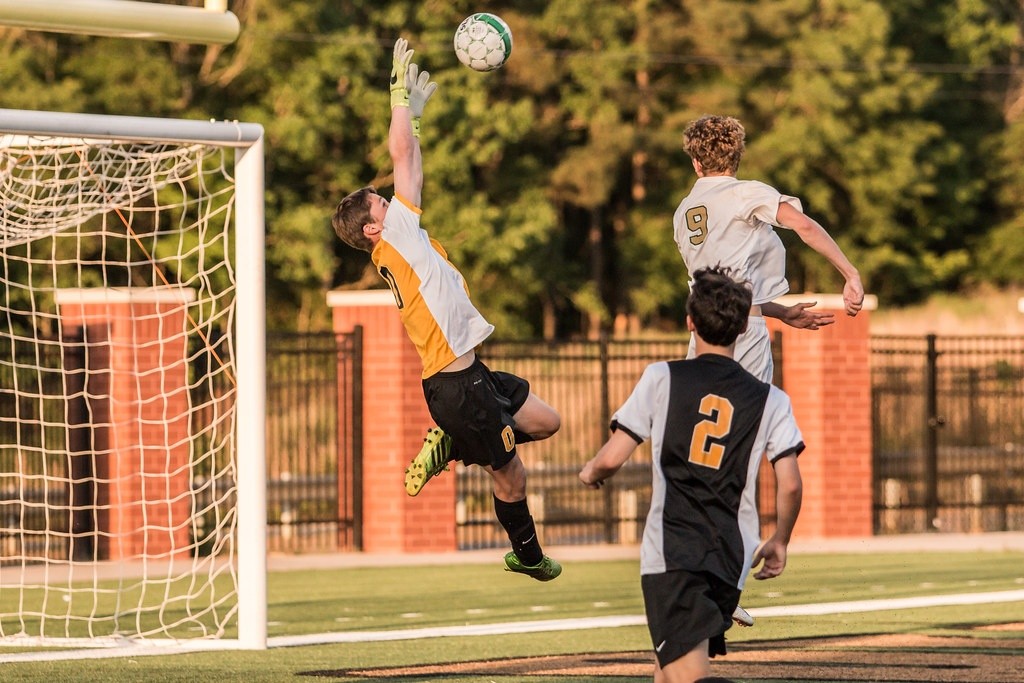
[503,550,562,582]
[732,604,754,627]
[404,426,453,497]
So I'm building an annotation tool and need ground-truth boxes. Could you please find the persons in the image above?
[329,35,562,582]
[579,261,805,683]
[671,115,865,626]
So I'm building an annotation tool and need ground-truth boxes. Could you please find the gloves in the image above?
[389,37,410,111]
[404,62,438,139]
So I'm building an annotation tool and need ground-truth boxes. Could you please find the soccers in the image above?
[453,12,514,73]
